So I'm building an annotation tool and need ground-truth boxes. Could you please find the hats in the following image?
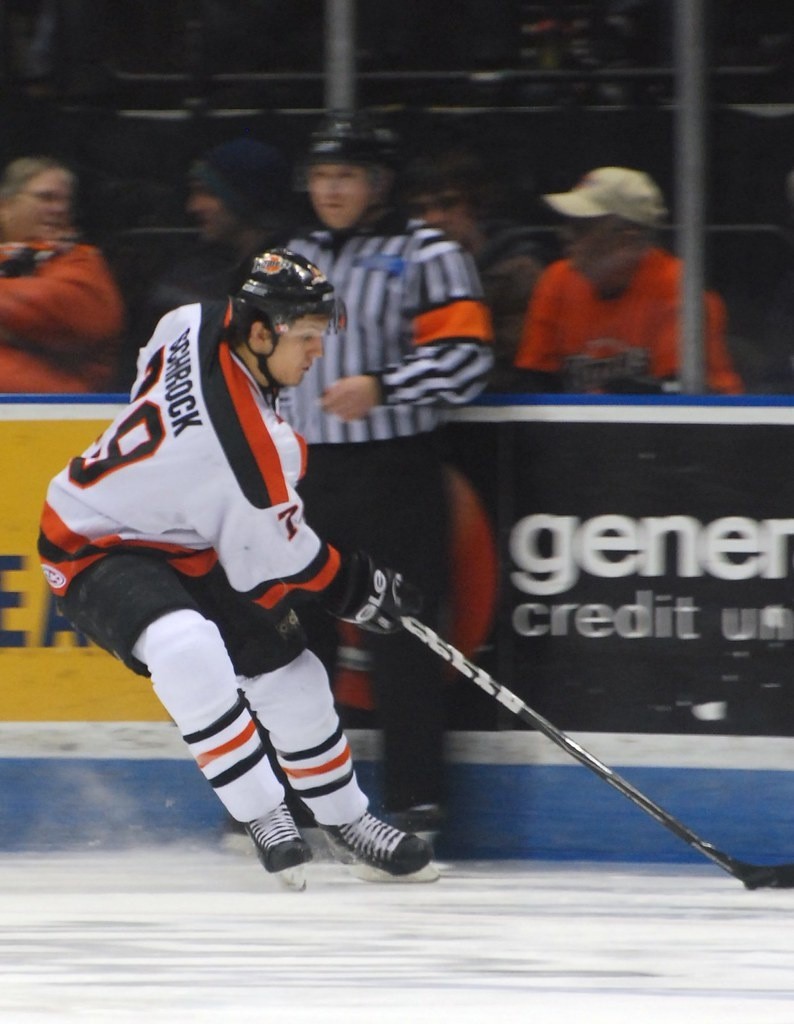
[542,166,668,229]
[204,137,285,208]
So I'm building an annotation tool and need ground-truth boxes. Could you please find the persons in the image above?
[216,111,492,862]
[38,254,444,894]
[188,140,746,394]
[0,155,127,395]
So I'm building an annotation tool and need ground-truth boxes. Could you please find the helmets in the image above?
[299,118,384,168]
[233,248,335,335]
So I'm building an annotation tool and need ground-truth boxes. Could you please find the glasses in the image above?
[402,191,469,219]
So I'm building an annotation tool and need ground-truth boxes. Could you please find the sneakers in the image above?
[222,798,325,855]
[381,813,443,843]
[244,799,313,891]
[316,811,439,882]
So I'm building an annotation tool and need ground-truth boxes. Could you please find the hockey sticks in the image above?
[396,606,794,890]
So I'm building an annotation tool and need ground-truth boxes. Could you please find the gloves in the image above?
[322,549,426,635]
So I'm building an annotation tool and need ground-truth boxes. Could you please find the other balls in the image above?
[744,868,775,889]
